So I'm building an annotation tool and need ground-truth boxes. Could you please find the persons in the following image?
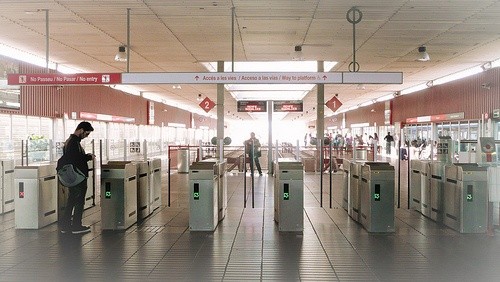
[243,132,262,174]
[384,131,393,155]
[373,133,380,154]
[61,121,96,234]
[304,133,307,146]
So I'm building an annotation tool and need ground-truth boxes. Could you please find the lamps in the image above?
[173,84,180,88]
[197,94,203,100]
[292,46,304,60]
[115,47,126,61]
[418,46,430,60]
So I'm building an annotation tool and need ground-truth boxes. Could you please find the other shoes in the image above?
[61,226,72,233]
[71,224,91,234]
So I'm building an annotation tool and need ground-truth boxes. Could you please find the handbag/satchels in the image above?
[257,150,261,157]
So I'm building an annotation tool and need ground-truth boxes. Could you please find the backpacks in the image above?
[56,154,85,194]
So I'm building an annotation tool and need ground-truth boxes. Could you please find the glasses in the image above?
[84,131,89,136]
